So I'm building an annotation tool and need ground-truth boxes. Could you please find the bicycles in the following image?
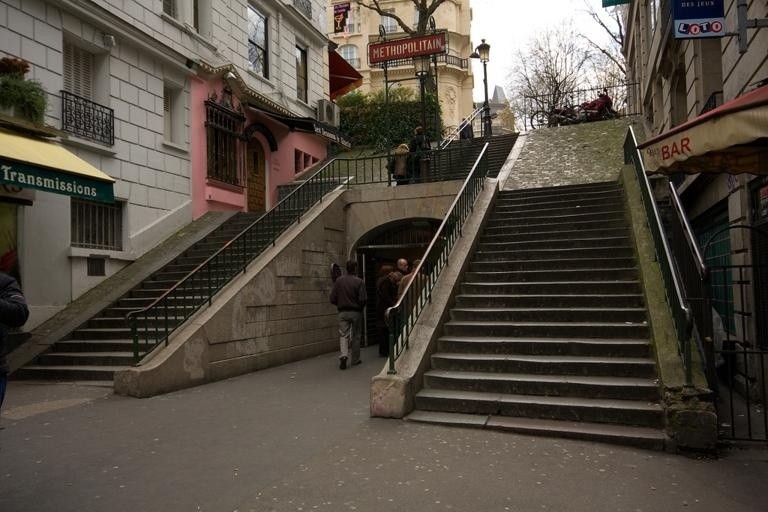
[531,93,587,130]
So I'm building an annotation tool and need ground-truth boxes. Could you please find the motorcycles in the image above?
[551,89,620,126]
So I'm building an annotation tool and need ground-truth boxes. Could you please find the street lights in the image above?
[470,45,479,60]
[469,39,497,136]
[413,55,432,183]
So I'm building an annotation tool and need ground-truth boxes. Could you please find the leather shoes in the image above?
[339,356,348,370]
[352,359,362,365]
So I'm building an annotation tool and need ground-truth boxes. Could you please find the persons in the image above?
[374,263,393,360]
[459,114,475,143]
[407,126,432,183]
[397,259,430,324]
[327,258,368,370]
[386,258,409,310]
[393,143,412,187]
[1,270,33,417]
